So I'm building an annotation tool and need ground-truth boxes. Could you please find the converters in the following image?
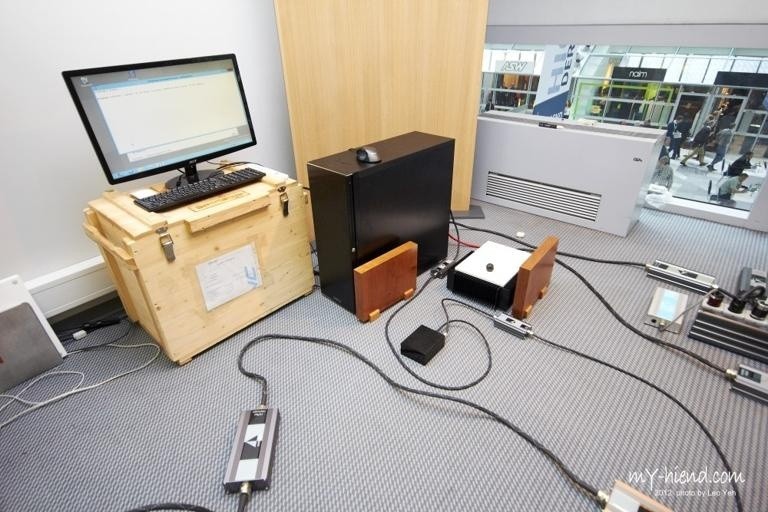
[644,287,688,334]
[732,364,768,402]
[494,312,532,340]
[431,258,457,279]
[647,259,715,294]
[223,408,279,493]
[401,325,445,366]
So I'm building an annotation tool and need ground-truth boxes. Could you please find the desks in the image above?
[740,163,766,190]
[730,188,757,211]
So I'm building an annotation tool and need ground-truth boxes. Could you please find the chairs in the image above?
[721,158,731,174]
[709,181,724,203]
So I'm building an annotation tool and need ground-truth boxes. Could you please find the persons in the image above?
[562,99,571,118]
[644,119,651,127]
[659,137,672,164]
[666,101,738,171]
[728,151,754,177]
[497,83,516,106]
[717,173,749,202]
[485,99,494,111]
[657,155,673,191]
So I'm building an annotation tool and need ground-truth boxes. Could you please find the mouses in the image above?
[356,146,381,163]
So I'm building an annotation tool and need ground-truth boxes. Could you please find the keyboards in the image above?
[134,167,266,212]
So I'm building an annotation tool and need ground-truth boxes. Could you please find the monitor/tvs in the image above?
[62,54,257,190]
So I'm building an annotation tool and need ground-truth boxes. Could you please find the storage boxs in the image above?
[81,161,316,366]
